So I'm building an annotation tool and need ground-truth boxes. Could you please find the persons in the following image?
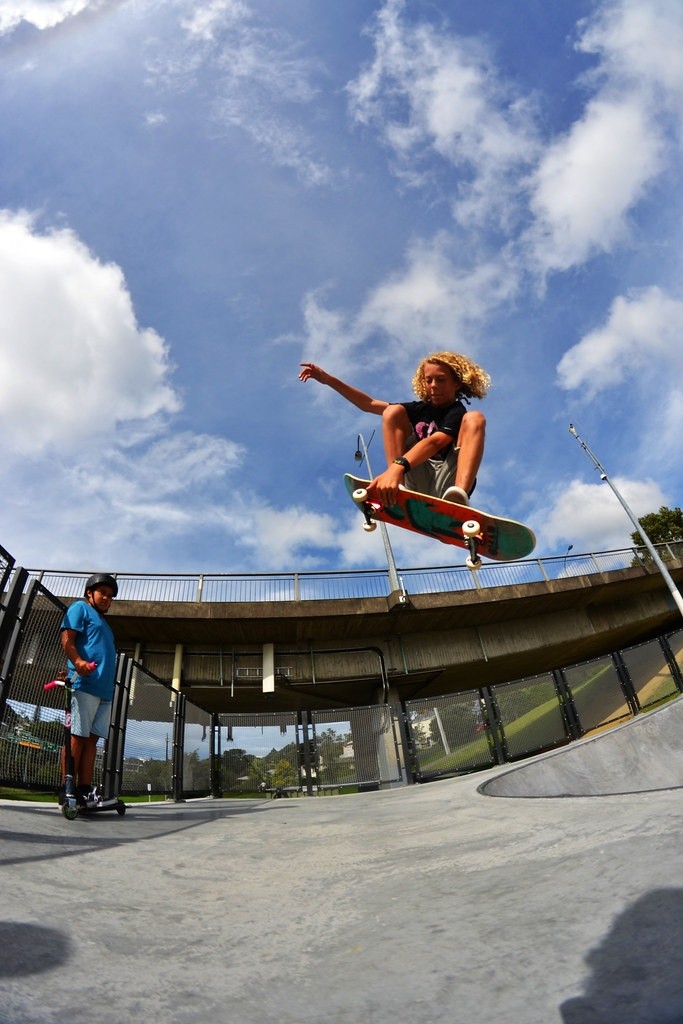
[59,574,118,802]
[298,351,491,505]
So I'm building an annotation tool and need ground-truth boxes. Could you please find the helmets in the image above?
[84,574,118,597]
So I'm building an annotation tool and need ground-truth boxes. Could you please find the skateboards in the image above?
[341,471,536,572]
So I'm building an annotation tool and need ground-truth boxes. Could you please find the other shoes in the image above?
[59,786,86,807]
[76,784,103,808]
[442,486,469,506]
[398,483,405,488]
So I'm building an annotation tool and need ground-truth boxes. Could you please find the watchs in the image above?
[393,457,411,474]
